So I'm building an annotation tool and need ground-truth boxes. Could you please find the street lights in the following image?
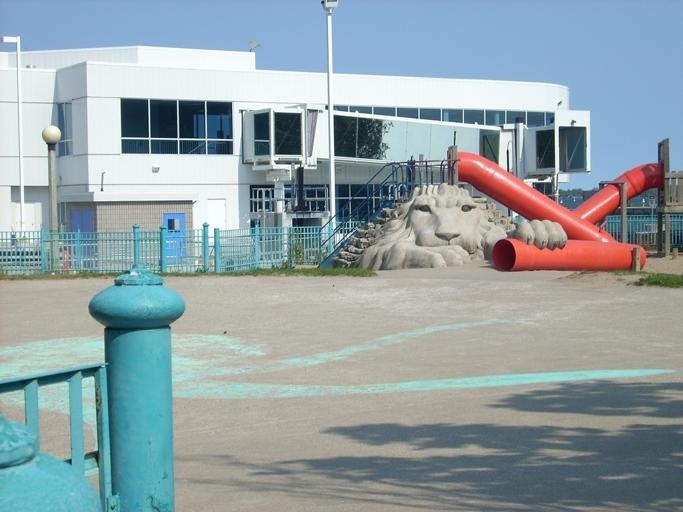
[41,125,61,273]
[320,1,339,256]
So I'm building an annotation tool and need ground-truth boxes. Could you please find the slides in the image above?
[318,201,392,269]
